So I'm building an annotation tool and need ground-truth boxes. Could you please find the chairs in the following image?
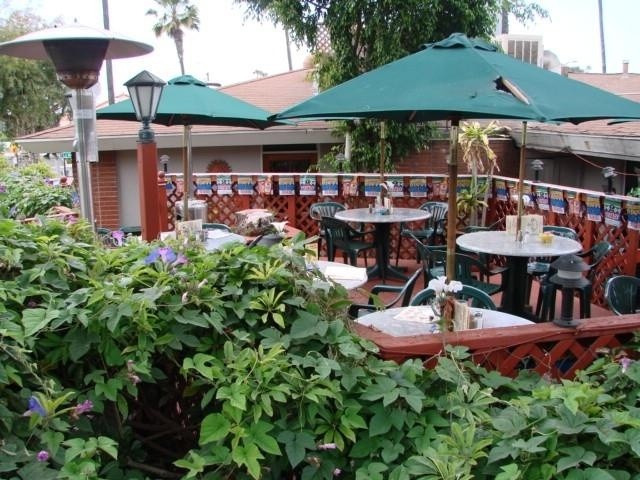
[395,202,448,267]
[321,216,376,266]
[467,227,507,284]
[411,286,497,310]
[429,250,508,307]
[535,243,612,323]
[605,275,640,315]
[544,226,578,240]
[410,235,462,285]
[310,202,367,266]
[349,269,423,319]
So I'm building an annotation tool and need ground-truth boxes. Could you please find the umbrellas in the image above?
[96,74,300,223]
[266,28,640,321]
